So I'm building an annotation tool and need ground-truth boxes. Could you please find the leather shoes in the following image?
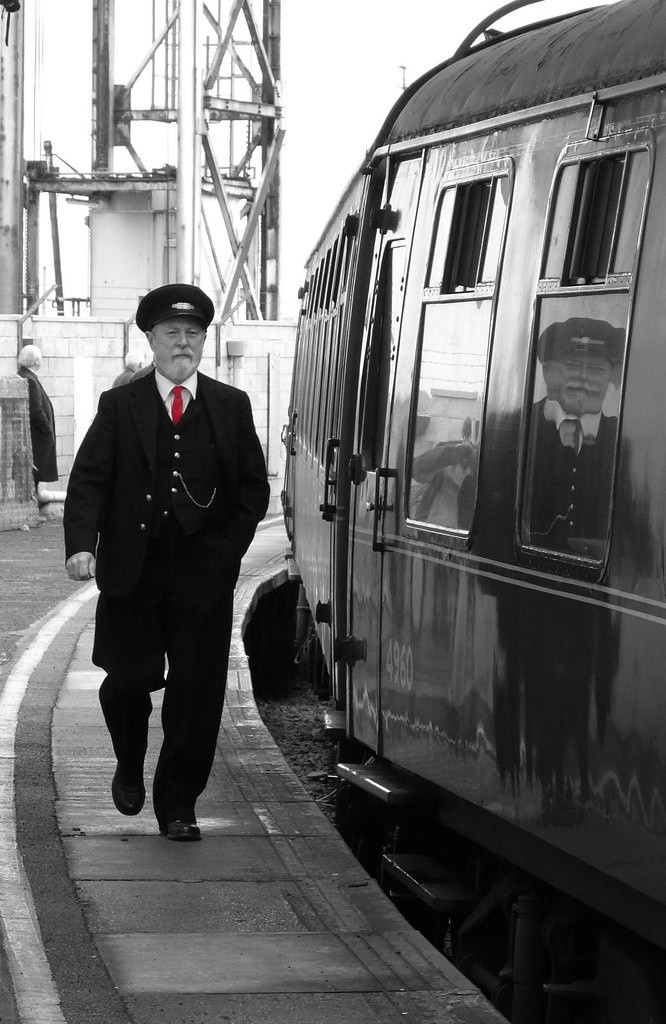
[161,817,201,840]
[111,759,146,816]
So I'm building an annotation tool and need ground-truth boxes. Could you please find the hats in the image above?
[537,317,626,364]
[136,284,214,333]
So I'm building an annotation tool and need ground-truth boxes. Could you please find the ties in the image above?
[574,420,580,455]
[172,386,186,426]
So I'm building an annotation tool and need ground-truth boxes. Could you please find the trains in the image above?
[278,0,665,1024]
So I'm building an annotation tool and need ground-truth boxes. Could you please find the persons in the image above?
[63,284,270,840]
[413,418,483,650]
[18,345,60,495]
[128,354,155,382]
[468,317,620,792]
[112,351,144,388]
